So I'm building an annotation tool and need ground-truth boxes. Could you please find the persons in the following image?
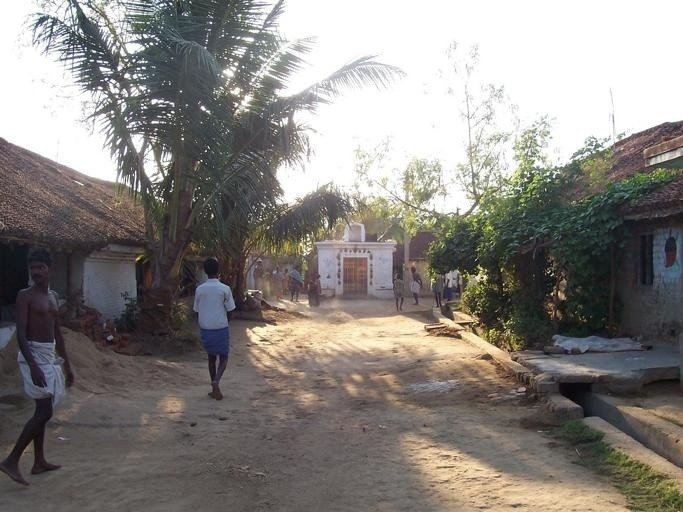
[253,259,320,309]
[443,270,452,301]
[410,266,422,305]
[433,275,441,306]
[192,255,236,398]
[0,249,75,485]
[392,272,404,312]
